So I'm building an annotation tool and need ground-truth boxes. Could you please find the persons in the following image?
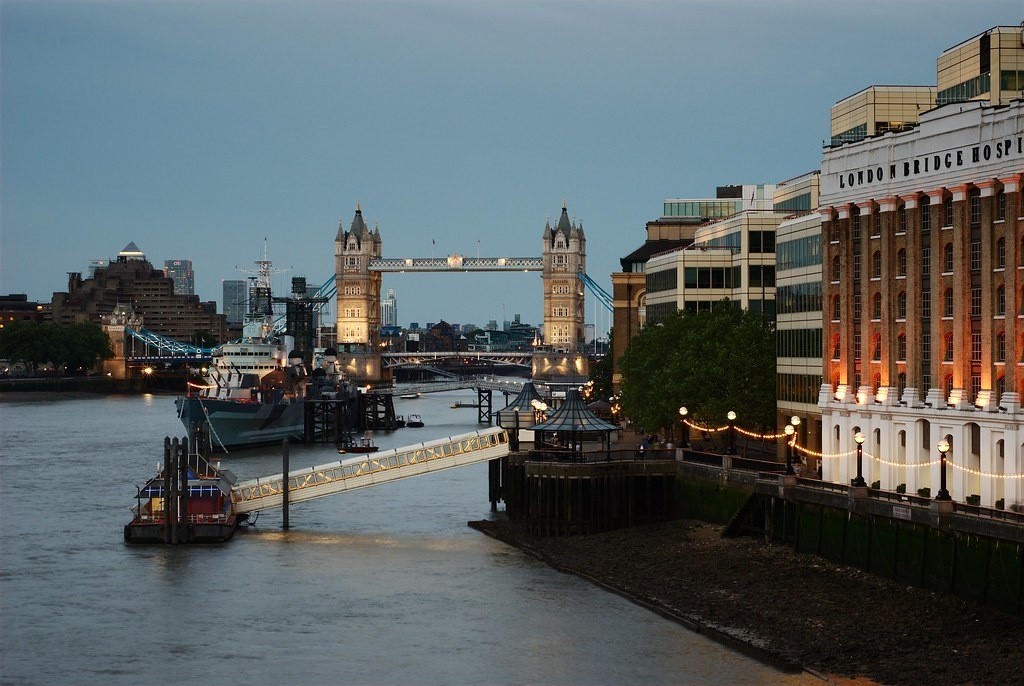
[491,361,496,383]
[250,384,279,404]
[344,347,351,353]
[456,344,460,352]
[464,380,480,388]
[404,388,409,393]
[551,433,580,453]
[473,399,476,408]
[527,371,533,381]
[125,349,129,357]
[1,368,111,379]
[548,349,553,354]
[204,405,208,414]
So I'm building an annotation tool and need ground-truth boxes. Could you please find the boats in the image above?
[394,414,407,428]
[123,430,249,546]
[336,437,380,453]
[406,413,426,428]
[173,272,369,457]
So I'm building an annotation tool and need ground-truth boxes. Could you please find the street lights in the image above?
[723,411,737,456]
[782,424,797,477]
[678,406,689,449]
[935,441,952,501]
[790,416,803,465]
[850,433,867,488]
